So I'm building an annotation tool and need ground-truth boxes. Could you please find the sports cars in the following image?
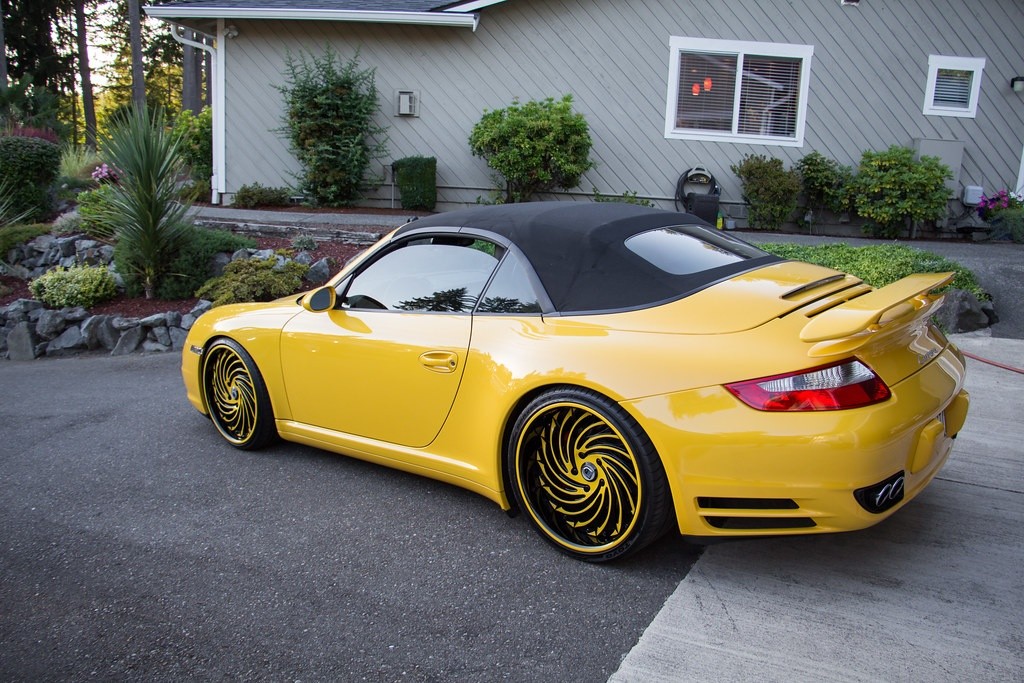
[178,200,970,565]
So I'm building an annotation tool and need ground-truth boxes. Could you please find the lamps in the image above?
[1011,76,1024,92]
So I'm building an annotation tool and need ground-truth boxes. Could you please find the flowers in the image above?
[975,187,1024,243]
